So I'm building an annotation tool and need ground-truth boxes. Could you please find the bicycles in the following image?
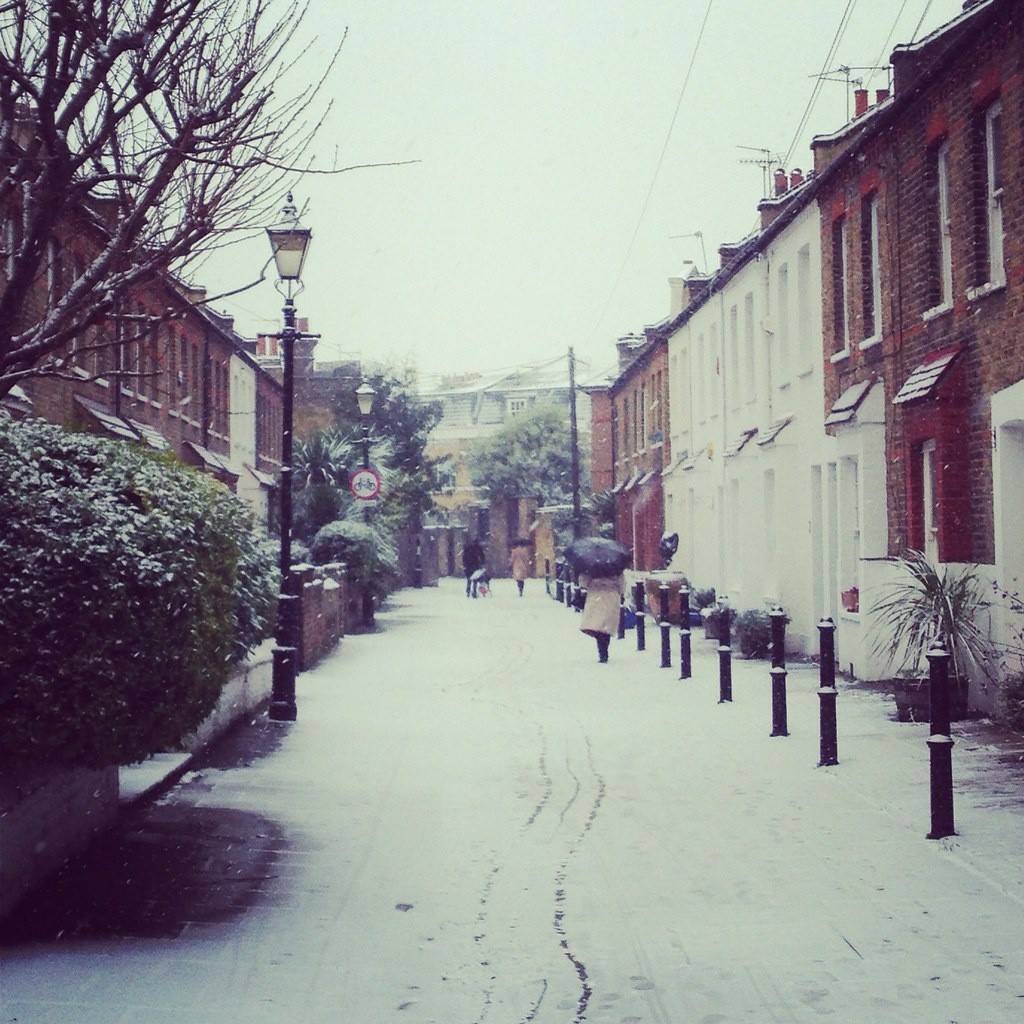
[474,564,493,598]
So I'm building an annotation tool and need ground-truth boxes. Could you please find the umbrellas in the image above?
[558,535,634,580]
[504,535,534,550]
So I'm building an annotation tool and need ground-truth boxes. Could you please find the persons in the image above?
[507,539,530,597]
[578,568,626,663]
[461,535,485,599]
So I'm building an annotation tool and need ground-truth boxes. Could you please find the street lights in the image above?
[259,192,325,723]
[353,373,380,629]
